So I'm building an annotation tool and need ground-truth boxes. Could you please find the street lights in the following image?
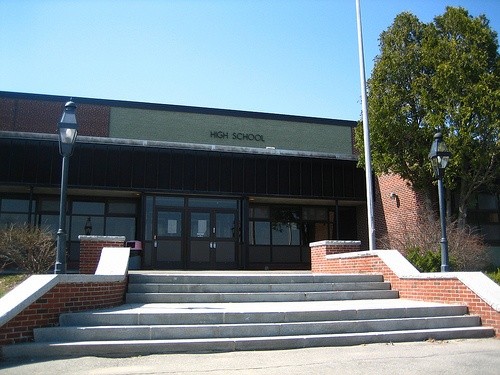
[54,101,78,275]
[430,132,451,272]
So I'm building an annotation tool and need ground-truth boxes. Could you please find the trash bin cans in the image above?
[125,240,144,269]
